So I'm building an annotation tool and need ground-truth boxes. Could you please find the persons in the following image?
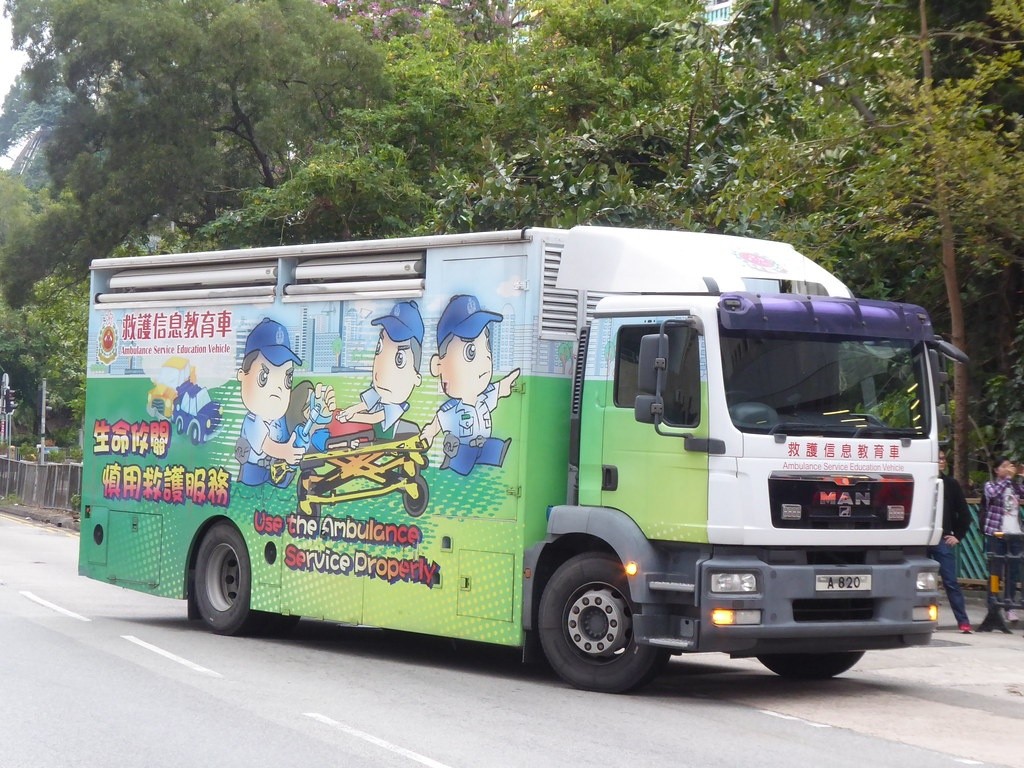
[35,429,55,454]
[984,457,1024,621]
[926,449,972,635]
[789,362,845,415]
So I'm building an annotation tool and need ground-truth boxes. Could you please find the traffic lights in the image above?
[5,386,22,412]
[37,390,54,416]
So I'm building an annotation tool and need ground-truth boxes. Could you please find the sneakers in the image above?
[958,623,973,634]
[1005,609,1019,622]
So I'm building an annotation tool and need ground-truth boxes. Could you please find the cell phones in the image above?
[1010,458,1017,467]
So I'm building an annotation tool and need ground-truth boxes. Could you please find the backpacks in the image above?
[977,490,988,532]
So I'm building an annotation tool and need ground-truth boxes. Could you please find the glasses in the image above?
[938,456,947,463]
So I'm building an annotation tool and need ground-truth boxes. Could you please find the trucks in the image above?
[80,224,973,695]
